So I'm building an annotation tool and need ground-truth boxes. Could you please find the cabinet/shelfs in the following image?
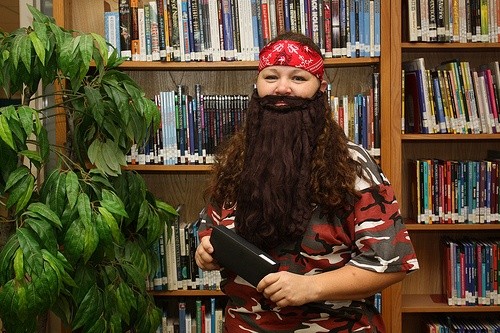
[390,0,500,333]
[54,0,390,333]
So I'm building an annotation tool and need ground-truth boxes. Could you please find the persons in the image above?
[194,28,421,333]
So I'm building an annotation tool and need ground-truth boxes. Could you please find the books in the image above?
[127,70,380,165]
[424,316,500,333]
[156,296,224,333]
[145,205,222,293]
[409,1,500,44]
[104,0,380,62]
[208,221,281,289]
[439,235,500,306]
[408,149,500,224]
[401,56,500,135]
